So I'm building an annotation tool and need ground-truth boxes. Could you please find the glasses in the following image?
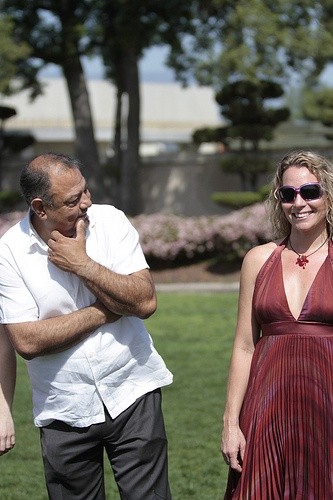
[279,182,323,203]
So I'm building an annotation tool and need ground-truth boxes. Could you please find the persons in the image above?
[0,151,174,500]
[220,151,333,500]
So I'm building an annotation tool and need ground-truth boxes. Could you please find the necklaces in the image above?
[288,233,329,271]
